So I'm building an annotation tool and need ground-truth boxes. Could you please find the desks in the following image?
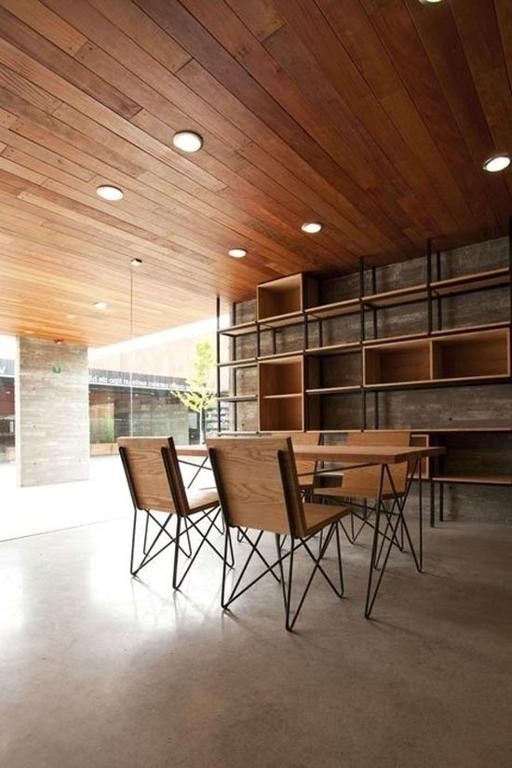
[173,444,445,620]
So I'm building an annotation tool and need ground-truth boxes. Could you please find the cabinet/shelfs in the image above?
[214,267,512,487]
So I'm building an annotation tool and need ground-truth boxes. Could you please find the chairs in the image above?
[117,432,413,630]
